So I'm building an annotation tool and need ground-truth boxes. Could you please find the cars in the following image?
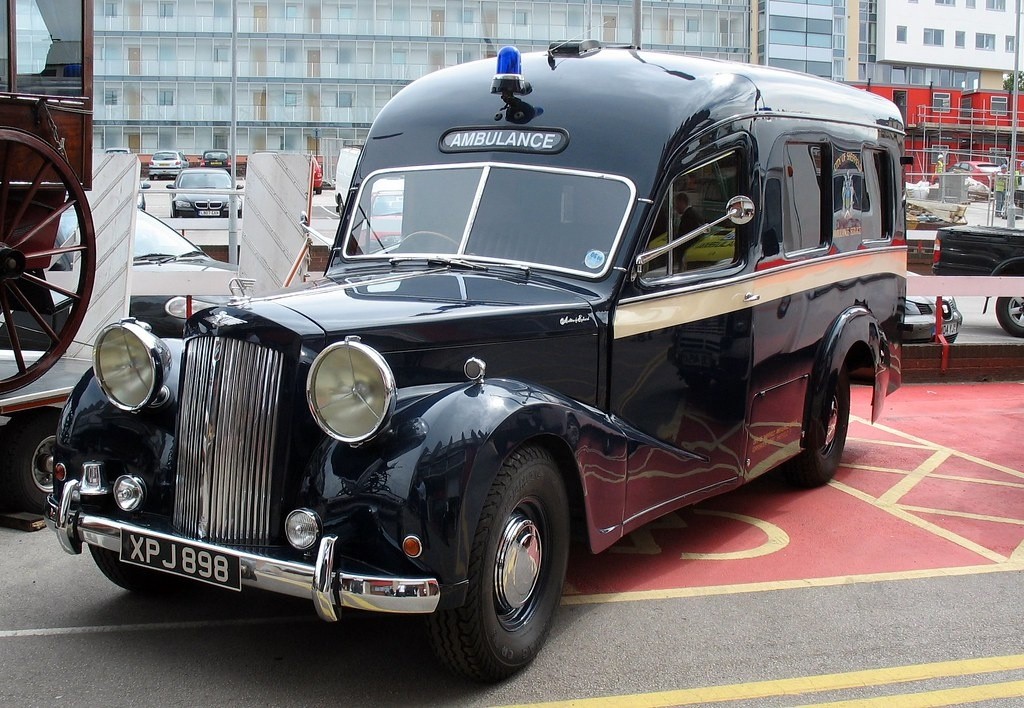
[930,160,1003,192]
[902,267,964,343]
[197,148,232,177]
[313,155,324,194]
[166,167,244,219]
[148,150,190,180]
[357,192,404,252]
[1,190,240,513]
[137,180,151,211]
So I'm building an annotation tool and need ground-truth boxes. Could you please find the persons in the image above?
[674,192,702,256]
[995,164,1008,217]
[1014,162,1024,220]
[935,154,943,174]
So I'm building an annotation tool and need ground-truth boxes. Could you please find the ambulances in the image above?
[42,37,914,689]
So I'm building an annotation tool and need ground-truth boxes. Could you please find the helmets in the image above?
[1001,164,1007,169]
[938,155,943,159]
[1020,162,1024,166]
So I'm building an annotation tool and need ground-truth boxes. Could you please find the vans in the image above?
[334,145,404,218]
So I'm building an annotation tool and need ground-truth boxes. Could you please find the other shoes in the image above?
[995,213,1002,217]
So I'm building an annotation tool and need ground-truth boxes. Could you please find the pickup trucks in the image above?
[932,224,1024,338]
